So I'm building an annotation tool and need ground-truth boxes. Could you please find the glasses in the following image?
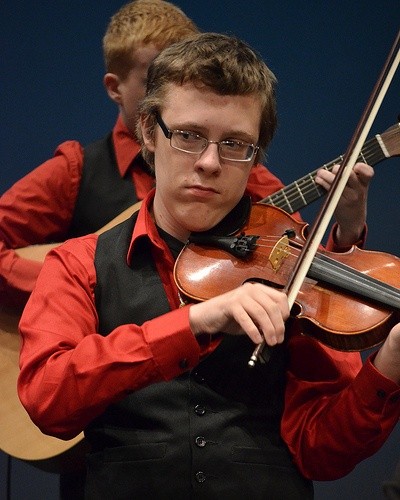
[156,115,259,161]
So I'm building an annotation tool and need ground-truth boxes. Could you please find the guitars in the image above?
[1,120,400,462]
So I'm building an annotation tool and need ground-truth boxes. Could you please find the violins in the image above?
[173,202,400,353]
